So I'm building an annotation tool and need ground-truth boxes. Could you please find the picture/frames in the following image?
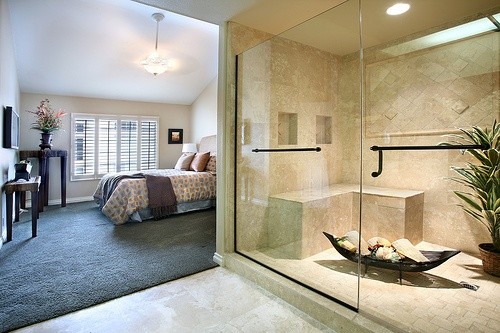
[167,128,184,144]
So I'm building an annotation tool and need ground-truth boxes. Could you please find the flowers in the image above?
[25,97,69,134]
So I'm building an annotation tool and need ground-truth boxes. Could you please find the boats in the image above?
[323,231,462,273]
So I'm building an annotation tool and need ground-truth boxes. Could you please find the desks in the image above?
[4,176,42,242]
[19,150,68,212]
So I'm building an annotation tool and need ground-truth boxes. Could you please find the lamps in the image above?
[139,13,173,75]
[182,143,197,153]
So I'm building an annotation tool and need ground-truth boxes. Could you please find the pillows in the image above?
[205,152,215,171]
[175,152,197,171]
[189,151,210,171]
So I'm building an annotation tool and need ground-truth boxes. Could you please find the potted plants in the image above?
[437,120,500,279]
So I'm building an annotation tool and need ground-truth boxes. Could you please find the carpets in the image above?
[0,200,220,333]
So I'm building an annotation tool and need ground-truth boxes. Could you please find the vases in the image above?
[39,132,54,151]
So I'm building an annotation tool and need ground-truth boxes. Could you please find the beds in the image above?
[92,168,216,225]
